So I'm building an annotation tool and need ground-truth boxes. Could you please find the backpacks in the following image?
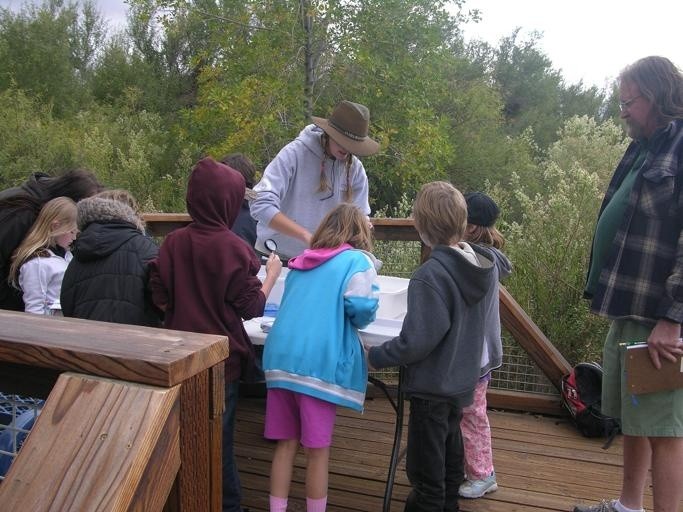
[558,361,622,451]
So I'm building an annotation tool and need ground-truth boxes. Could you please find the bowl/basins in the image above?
[371,275,411,319]
[256,264,292,312]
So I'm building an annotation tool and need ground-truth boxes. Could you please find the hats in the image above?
[311,100,381,156]
[461,192,500,227]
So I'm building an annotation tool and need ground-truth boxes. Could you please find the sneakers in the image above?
[571,501,646,512]
[459,471,498,499]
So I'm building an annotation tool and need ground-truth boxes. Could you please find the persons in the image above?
[456,192,514,499]
[219,153,259,257]
[260,202,386,512]
[6,196,78,316]
[247,100,379,270]
[362,179,498,512]
[571,54,683,512]
[59,187,163,330]
[145,156,284,512]
[1,167,102,313]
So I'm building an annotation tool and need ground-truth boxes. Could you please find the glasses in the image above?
[618,94,643,111]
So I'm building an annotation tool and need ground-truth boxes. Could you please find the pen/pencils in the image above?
[625,344,652,350]
[617,341,651,345]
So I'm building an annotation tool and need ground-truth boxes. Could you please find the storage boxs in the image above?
[379,275,410,319]
[257,264,291,305]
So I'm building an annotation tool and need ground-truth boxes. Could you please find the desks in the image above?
[242,315,407,512]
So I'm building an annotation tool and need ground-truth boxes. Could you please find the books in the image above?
[626,342,683,397]
